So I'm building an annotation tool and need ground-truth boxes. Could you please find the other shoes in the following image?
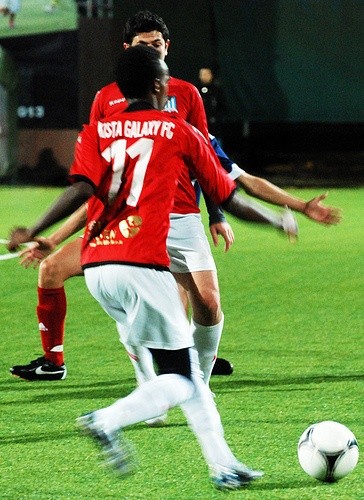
[210,468,264,492]
[78,413,131,476]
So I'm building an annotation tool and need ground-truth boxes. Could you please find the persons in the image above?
[6,43,267,490]
[92,12,236,428]
[8,129,344,383]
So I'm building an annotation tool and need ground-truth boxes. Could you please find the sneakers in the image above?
[211,359,232,375]
[9,356,66,381]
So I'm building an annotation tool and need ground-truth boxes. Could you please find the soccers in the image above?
[298,420,359,482]
